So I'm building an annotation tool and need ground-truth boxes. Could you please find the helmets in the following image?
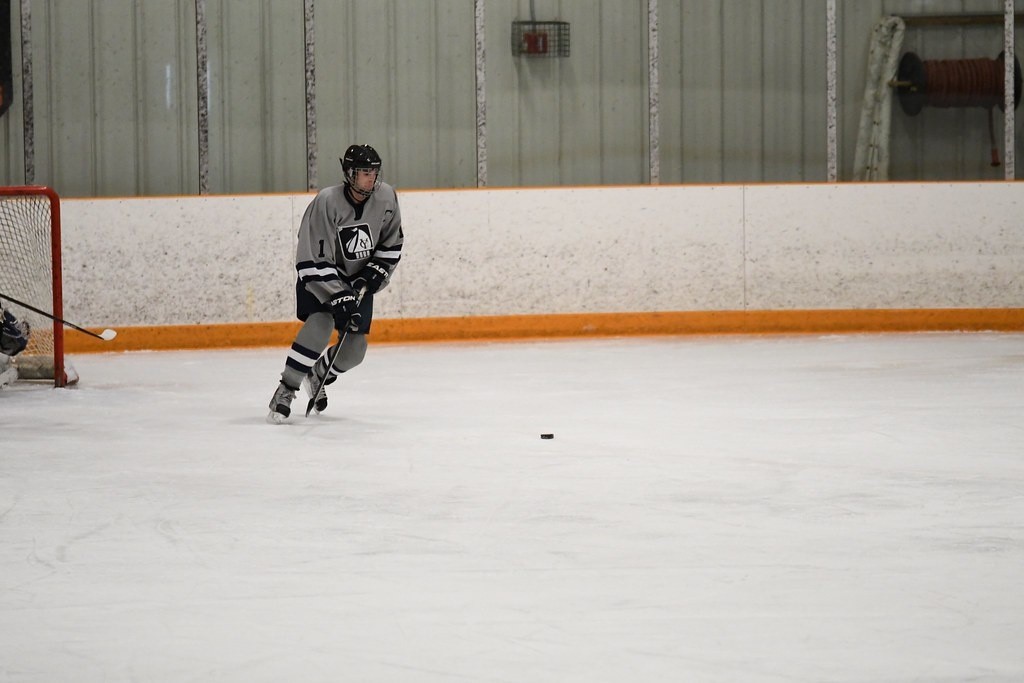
[338,144,383,198]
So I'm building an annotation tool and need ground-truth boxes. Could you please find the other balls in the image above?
[540,433,555,440]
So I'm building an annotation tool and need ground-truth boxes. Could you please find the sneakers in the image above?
[309,366,329,412]
[269,379,297,419]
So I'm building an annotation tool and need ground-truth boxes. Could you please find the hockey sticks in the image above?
[0,293,118,341]
[304,284,369,417]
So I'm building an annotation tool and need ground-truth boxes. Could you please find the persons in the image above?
[267,144,404,418]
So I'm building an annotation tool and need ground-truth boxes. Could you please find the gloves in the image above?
[348,257,391,295]
[329,290,362,336]
